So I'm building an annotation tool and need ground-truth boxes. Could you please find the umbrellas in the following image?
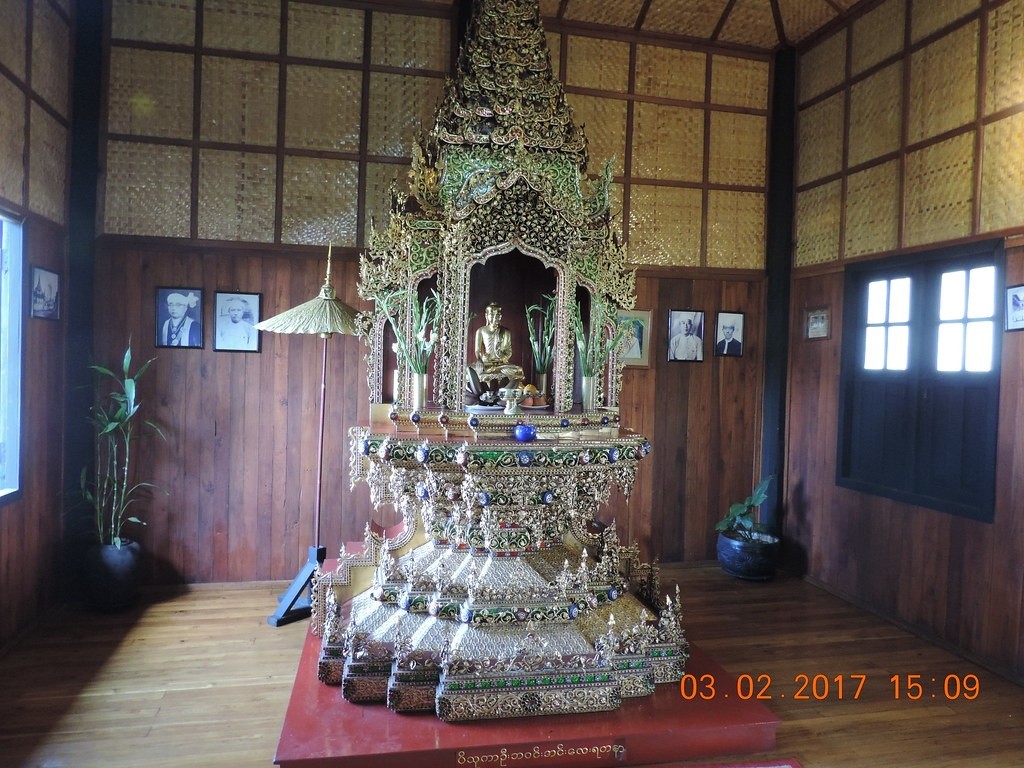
[249,239,374,545]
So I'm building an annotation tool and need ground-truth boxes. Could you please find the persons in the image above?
[474,301,524,382]
[671,313,703,361]
[218,298,253,345]
[617,321,640,359]
[717,319,741,354]
[158,292,201,346]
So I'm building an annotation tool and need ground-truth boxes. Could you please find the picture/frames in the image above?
[611,308,654,371]
[667,308,705,362]
[713,310,745,357]
[1005,284,1024,331]
[153,286,204,349]
[28,264,63,321]
[802,303,831,341]
[213,290,261,352]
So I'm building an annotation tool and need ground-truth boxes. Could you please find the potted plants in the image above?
[564,291,646,412]
[715,476,781,581]
[525,292,557,395]
[373,287,443,411]
[77,331,169,607]
